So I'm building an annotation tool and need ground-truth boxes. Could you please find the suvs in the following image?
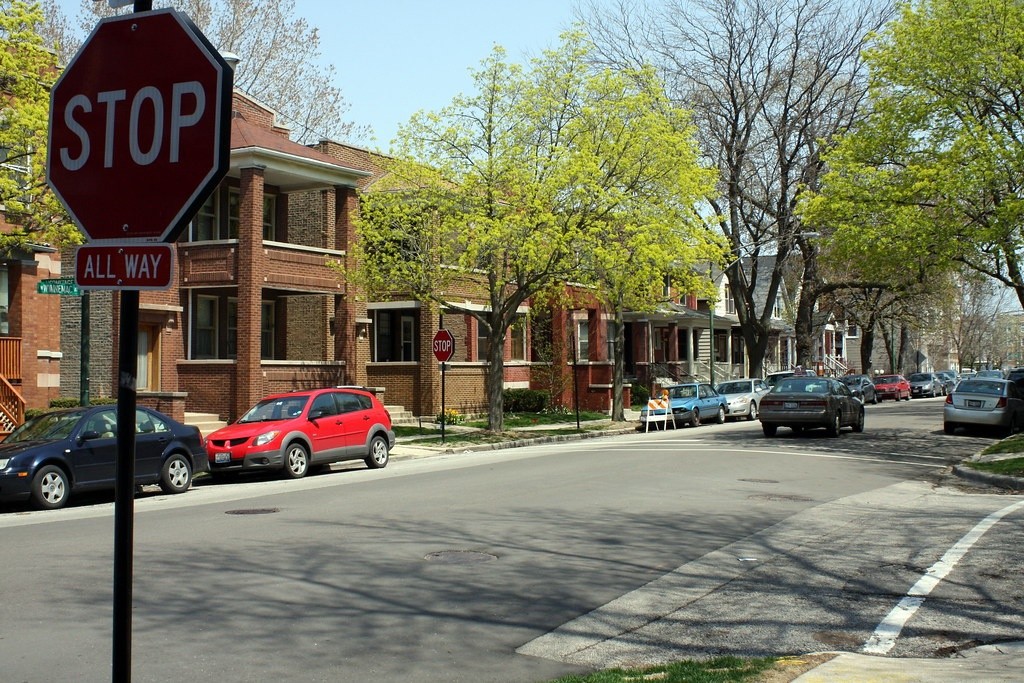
[205,385,396,481]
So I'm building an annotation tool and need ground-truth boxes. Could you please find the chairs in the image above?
[139,420,155,434]
[727,387,742,392]
[782,388,791,391]
[287,405,301,415]
[674,390,683,397]
[812,387,822,394]
[94,420,114,439]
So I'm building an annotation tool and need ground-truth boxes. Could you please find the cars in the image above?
[759,376,864,438]
[0,403,211,511]
[713,378,771,421]
[639,382,731,427]
[835,375,878,407]
[872,373,911,402]
[909,368,1024,399]
[942,377,1024,438]
[760,369,818,391]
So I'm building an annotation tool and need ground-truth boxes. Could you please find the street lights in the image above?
[708,231,820,393]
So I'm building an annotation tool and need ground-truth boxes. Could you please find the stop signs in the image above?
[47,6,234,248]
[433,329,456,363]
